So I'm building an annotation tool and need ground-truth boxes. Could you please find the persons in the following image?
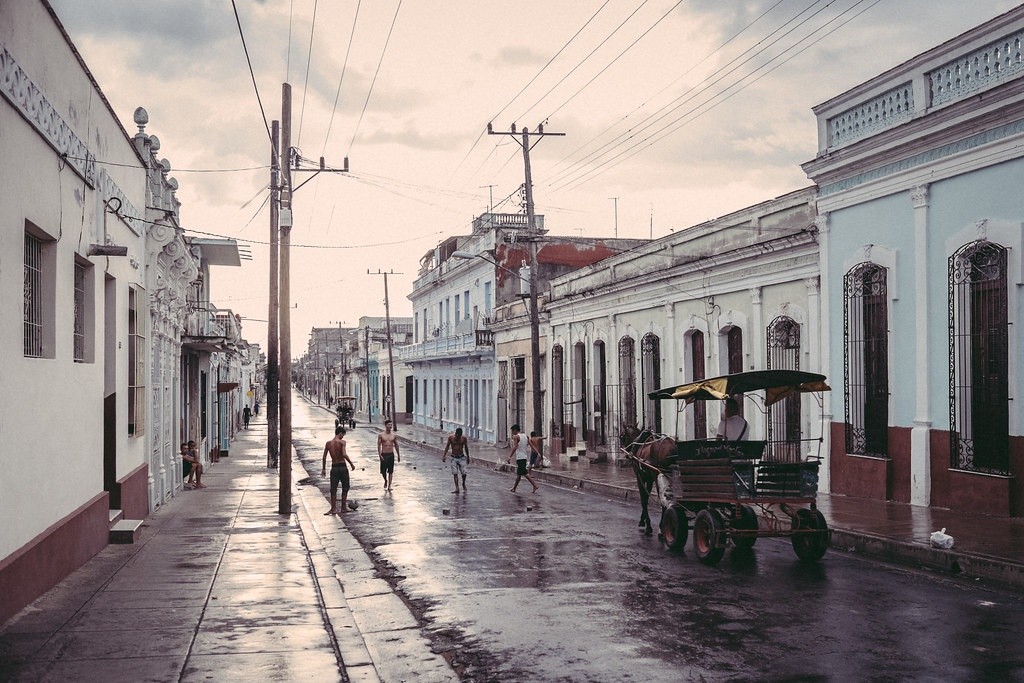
[253,402,260,416]
[244,404,251,429]
[378,420,400,490]
[716,399,751,463]
[181,441,207,488]
[442,428,470,493]
[507,424,541,493]
[527,432,548,476]
[321,426,355,515]
[335,401,348,413]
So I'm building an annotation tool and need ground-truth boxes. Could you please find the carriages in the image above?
[335,396,358,429]
[618,370,834,564]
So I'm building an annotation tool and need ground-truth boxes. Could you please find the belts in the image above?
[451,454,465,459]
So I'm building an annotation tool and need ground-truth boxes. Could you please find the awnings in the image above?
[219,382,240,394]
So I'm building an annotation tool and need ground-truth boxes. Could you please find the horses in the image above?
[619,421,678,540]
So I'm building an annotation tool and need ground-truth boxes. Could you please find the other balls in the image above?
[348,499,359,509]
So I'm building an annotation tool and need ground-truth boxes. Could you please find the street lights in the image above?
[451,250,544,467]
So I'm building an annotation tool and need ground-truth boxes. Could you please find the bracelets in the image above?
[508,457,511,459]
